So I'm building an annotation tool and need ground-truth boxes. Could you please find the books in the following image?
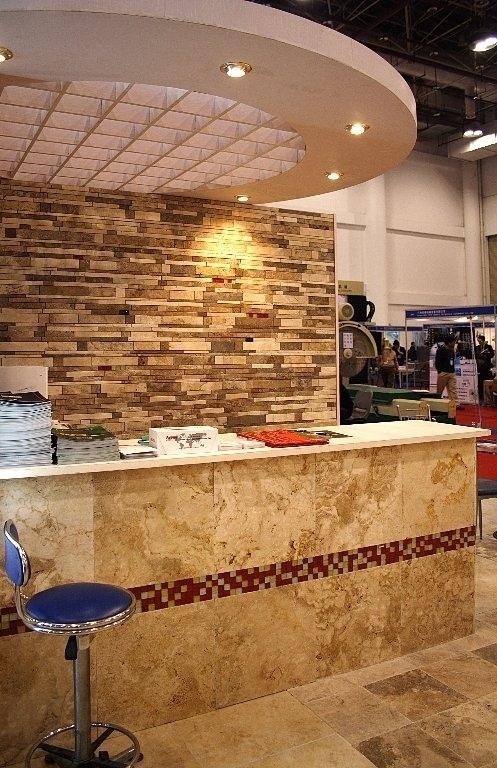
[2,390,158,466]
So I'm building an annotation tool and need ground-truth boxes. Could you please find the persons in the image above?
[340,384,355,422]
[368,335,495,410]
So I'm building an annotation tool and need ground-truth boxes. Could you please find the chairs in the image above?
[1,517,142,767]
[343,388,373,425]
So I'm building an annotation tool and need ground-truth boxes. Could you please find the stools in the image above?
[477,476,497,539]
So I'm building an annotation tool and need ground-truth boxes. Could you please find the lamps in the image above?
[467,27,496,53]
[459,120,483,138]
